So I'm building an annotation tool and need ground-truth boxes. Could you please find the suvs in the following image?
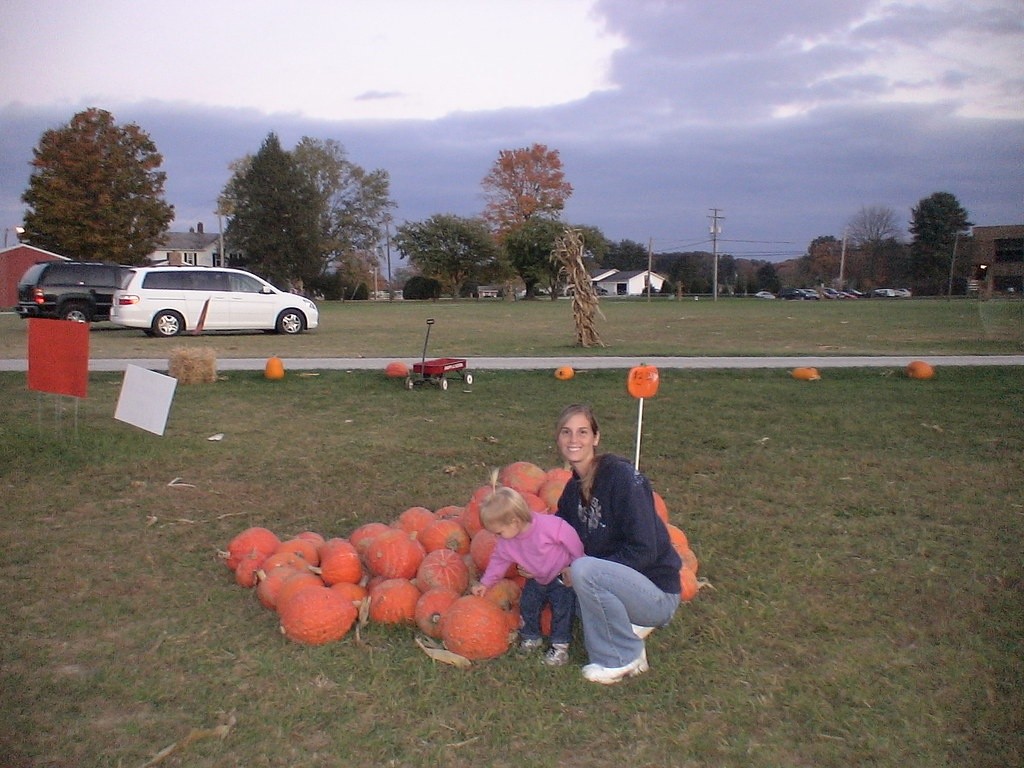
[15,261,130,322]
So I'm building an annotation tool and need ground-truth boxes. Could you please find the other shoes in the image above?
[540,643,569,666]
[519,639,542,649]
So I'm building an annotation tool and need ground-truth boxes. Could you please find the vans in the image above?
[111,268,319,335]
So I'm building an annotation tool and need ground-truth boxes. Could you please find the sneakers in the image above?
[630,623,656,640]
[582,648,649,685]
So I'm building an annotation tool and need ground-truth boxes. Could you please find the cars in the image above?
[874,288,910,298]
[785,288,870,300]
[756,291,775,300]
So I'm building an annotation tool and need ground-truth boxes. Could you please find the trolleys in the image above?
[406,319,473,391]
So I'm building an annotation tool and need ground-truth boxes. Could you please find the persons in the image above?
[515,403,684,686]
[470,468,585,667]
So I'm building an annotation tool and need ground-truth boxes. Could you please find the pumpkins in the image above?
[555,364,574,380]
[792,367,818,380]
[264,354,284,379]
[224,461,699,661]
[384,361,409,378]
[904,360,933,379]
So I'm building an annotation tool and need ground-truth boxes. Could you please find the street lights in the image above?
[5,227,25,250]
